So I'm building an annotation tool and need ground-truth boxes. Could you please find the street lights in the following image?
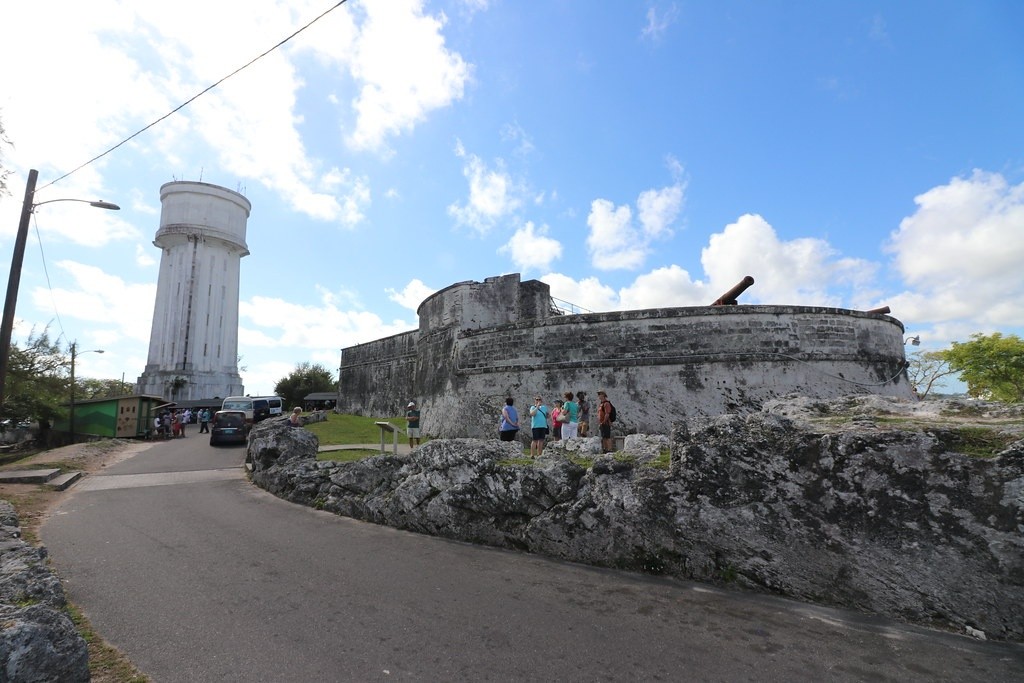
[0,198,120,404]
[70,350,104,444]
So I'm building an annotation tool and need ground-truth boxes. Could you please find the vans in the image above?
[221,396,286,429]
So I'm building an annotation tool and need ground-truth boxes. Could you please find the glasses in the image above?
[536,398,541,401]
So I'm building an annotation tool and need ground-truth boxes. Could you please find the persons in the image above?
[499,397,520,442]
[289,406,306,427]
[406,402,422,451]
[197,408,210,424]
[576,391,590,437]
[185,408,193,424]
[596,389,616,454]
[560,391,579,439]
[152,409,187,438]
[551,399,565,441]
[198,408,209,434]
[530,395,549,460]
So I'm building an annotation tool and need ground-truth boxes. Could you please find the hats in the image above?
[596,388,606,395]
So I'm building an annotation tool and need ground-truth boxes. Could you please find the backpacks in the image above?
[602,400,616,422]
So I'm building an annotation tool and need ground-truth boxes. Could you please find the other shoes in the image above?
[531,455,535,459]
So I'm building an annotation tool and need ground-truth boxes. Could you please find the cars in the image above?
[210,410,247,445]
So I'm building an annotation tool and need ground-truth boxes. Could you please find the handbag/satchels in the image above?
[556,410,569,423]
[545,425,549,434]
